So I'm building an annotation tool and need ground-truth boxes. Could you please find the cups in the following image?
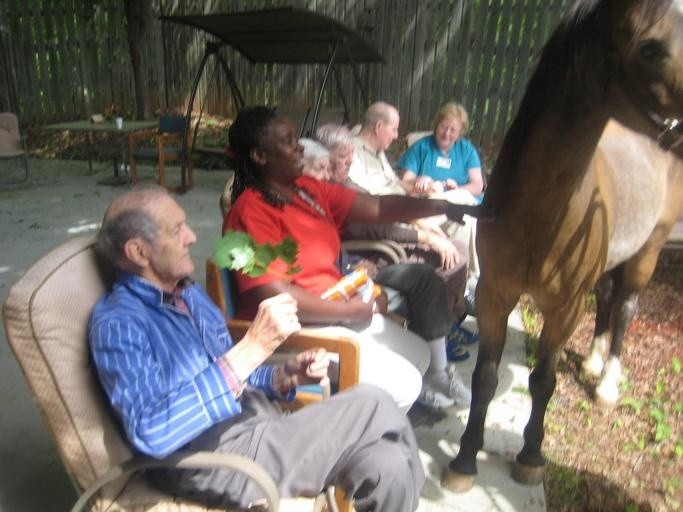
[115,117,124,131]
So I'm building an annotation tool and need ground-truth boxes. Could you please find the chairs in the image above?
[0,112,31,190]
[204,244,385,512]
[407,130,435,146]
[4,234,342,512]
[128,113,194,194]
[220,173,401,265]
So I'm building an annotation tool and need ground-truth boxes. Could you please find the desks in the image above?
[40,118,160,187]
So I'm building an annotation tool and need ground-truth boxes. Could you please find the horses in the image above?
[439,0,683,494]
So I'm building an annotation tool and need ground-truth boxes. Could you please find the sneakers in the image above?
[417,291,478,409]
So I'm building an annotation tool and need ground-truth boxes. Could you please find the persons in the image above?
[223,102,483,415]
[87,182,426,512]
